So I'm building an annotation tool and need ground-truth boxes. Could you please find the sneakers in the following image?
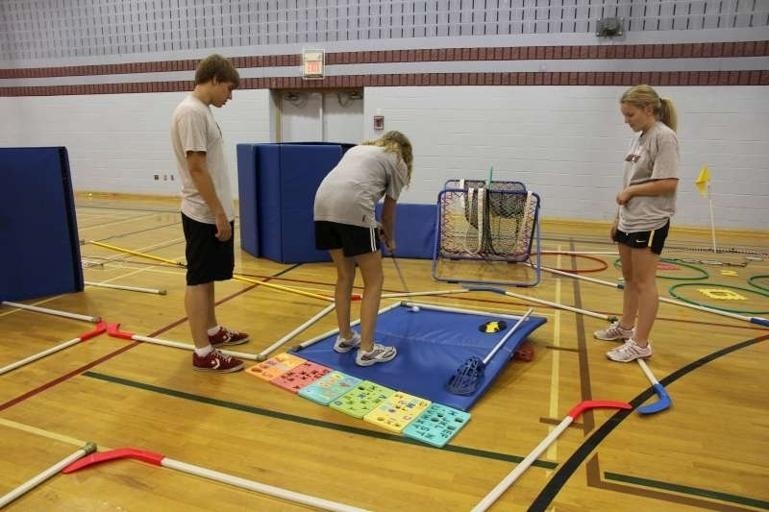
[604,337,654,363]
[595,321,636,342]
[333,330,364,355]
[354,343,396,367]
[205,325,250,346]
[190,347,245,374]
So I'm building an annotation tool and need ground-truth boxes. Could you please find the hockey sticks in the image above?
[61,445,364,511]
[468,284,615,323]
[469,400,632,511]
[635,356,672,413]
[106,320,260,360]
[0,317,105,382]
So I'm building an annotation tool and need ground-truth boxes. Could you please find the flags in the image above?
[695,166,709,194]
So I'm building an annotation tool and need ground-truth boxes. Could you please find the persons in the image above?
[312,130,413,367]
[592,85,681,363]
[169,56,251,374]
